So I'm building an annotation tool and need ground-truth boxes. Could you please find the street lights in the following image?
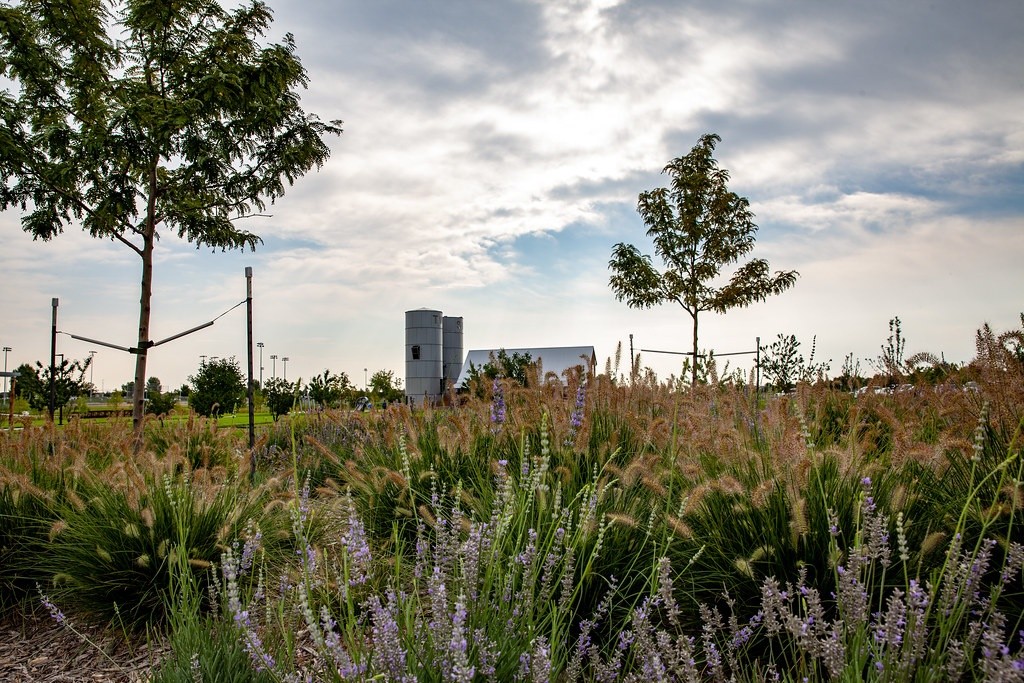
[364,368,368,391]
[270,355,278,389]
[281,357,290,386]
[89,350,98,401]
[256,342,266,395]
[200,355,207,368]
[2,345,13,406]
[55,354,65,427]
